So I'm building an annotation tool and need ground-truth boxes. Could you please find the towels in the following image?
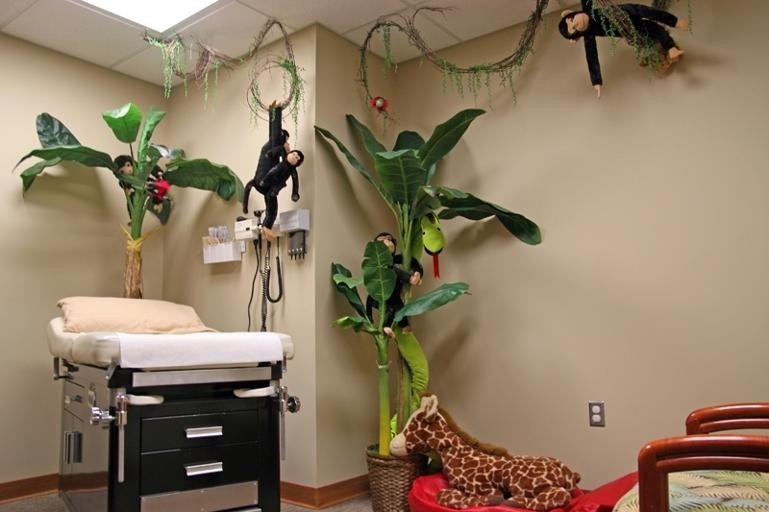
[98,330,284,369]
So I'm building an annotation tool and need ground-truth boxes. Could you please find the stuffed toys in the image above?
[235,100,302,239]
[387,391,582,511]
[114,153,170,207]
[557,0,686,97]
[410,206,446,281]
[364,230,418,340]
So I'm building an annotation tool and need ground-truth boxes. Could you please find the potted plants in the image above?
[312,109,544,512]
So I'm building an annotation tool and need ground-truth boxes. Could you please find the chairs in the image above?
[569,404,768,512]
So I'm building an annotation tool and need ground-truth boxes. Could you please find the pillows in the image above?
[56,295,214,335]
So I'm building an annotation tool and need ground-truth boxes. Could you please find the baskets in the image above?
[366,444,428,512]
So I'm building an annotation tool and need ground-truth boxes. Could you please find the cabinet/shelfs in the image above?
[57,360,281,512]
